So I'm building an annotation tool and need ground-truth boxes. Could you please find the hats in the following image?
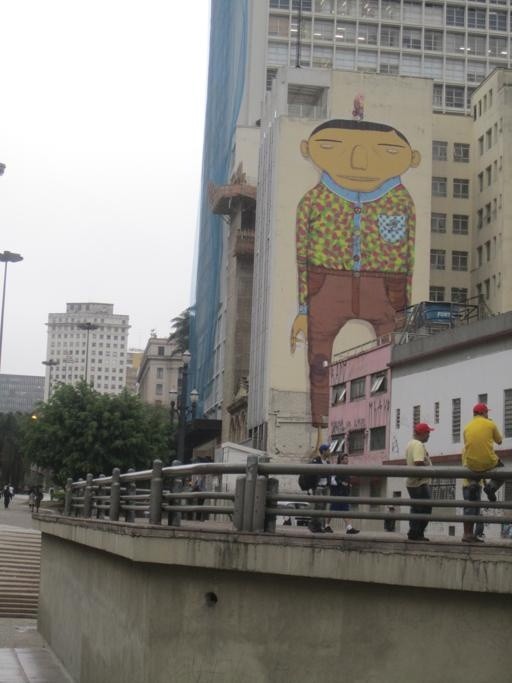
[318,444,332,454]
[413,422,435,434]
[473,402,494,414]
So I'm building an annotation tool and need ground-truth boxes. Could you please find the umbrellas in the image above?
[31,485,44,489]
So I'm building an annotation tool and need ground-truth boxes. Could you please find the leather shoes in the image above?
[462,534,485,543]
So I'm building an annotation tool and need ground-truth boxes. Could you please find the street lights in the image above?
[169,349,200,465]
[0,251,23,362]
[78,321,100,381]
[42,360,59,402]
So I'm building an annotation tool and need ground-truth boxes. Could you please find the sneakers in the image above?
[484,483,498,501]
[469,483,479,500]
[407,529,429,541]
[311,526,360,534]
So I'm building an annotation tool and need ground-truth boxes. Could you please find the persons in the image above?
[0,483,14,510]
[34,489,43,514]
[462,402,510,504]
[48,484,55,500]
[28,489,35,512]
[404,423,436,541]
[458,450,486,543]
[308,443,332,534]
[323,450,361,535]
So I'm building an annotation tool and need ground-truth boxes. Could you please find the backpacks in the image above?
[298,458,322,491]
